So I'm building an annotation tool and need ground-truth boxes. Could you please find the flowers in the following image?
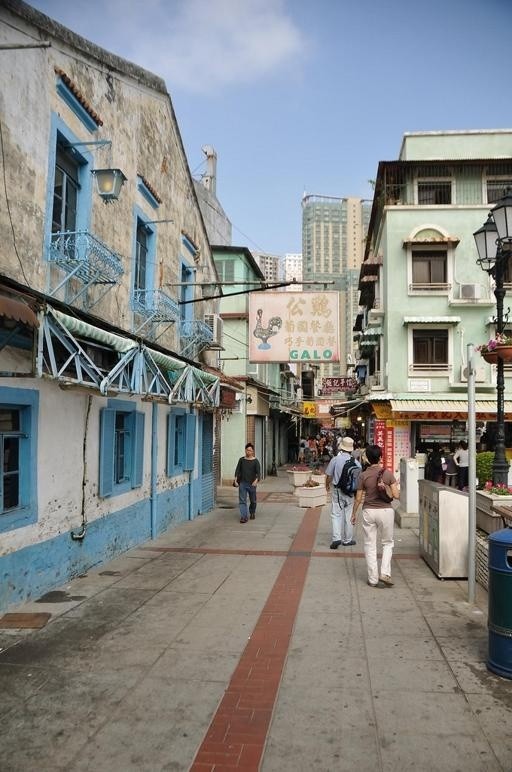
[286,465,308,471]
[475,333,512,353]
[303,479,319,487]
[314,468,321,474]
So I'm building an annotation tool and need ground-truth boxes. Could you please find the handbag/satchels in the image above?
[232,472,241,487]
[377,477,393,503]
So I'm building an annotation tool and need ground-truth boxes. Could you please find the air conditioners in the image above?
[460,365,486,382]
[459,282,481,299]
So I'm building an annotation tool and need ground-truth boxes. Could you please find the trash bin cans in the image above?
[488,527,512,680]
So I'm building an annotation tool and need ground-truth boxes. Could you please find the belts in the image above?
[333,485,340,488]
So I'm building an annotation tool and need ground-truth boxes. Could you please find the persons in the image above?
[233,443,260,523]
[325,436,399,587]
[419,441,468,492]
[288,431,371,472]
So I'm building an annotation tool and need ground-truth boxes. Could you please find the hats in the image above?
[340,436,354,452]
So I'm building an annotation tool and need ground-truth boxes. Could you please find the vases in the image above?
[287,470,312,487]
[294,486,327,509]
[483,346,512,363]
[311,473,327,489]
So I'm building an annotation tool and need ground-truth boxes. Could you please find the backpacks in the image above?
[300,443,305,448]
[337,457,362,497]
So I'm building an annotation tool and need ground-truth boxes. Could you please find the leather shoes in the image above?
[330,539,357,549]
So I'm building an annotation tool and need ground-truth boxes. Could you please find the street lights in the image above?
[471,181,512,491]
[269,395,281,477]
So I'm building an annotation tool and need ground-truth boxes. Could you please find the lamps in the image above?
[64,141,127,203]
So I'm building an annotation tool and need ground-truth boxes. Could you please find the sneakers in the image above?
[240,511,256,523]
[367,574,395,587]
